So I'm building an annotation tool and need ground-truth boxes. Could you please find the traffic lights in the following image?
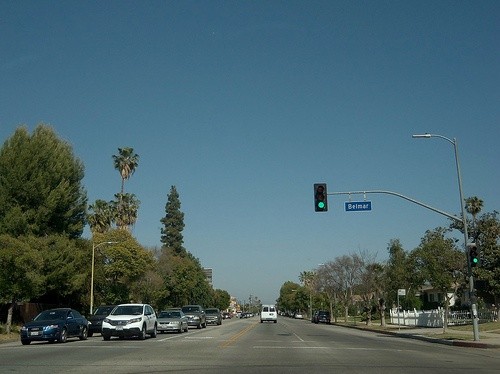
[467,242,478,267]
[314,183,328,212]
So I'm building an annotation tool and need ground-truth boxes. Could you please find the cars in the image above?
[86,304,117,337]
[278,310,304,319]
[156,309,188,334]
[221,310,260,320]
[20,310,90,345]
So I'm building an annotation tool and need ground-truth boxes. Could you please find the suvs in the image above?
[203,307,222,326]
[181,304,206,329]
[101,303,158,340]
[312,310,331,325]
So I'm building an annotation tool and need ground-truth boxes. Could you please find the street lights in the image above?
[412,133,479,342]
[90,241,118,315]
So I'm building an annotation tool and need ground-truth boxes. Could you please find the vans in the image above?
[259,304,278,323]
